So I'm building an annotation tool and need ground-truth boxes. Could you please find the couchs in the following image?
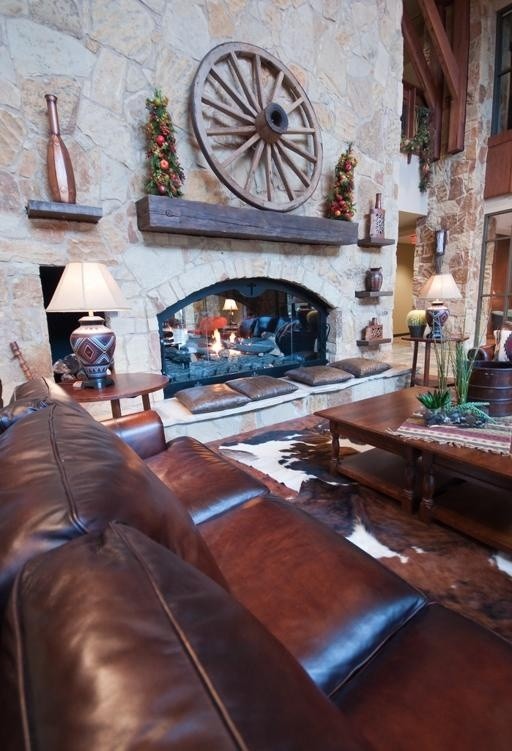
[0,382,512,655]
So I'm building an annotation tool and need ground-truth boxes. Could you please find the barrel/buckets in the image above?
[464,360,512,416]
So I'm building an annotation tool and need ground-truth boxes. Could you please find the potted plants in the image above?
[405,309,427,337]
[412,306,492,431]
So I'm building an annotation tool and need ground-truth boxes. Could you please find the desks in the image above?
[54,370,172,421]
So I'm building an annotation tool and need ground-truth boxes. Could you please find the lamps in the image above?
[418,273,463,338]
[432,229,450,272]
[41,261,134,389]
[220,298,239,327]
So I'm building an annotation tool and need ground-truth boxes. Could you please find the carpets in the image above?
[205,415,511,638]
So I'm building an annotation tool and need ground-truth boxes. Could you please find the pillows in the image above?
[175,356,389,418]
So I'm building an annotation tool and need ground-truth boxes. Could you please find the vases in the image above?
[368,192,388,238]
[365,262,383,292]
[42,90,83,203]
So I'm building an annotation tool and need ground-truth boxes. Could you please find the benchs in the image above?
[153,352,419,440]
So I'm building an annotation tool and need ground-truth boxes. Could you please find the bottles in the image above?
[366,266,383,291]
[364,317,383,340]
[368,191,384,239]
